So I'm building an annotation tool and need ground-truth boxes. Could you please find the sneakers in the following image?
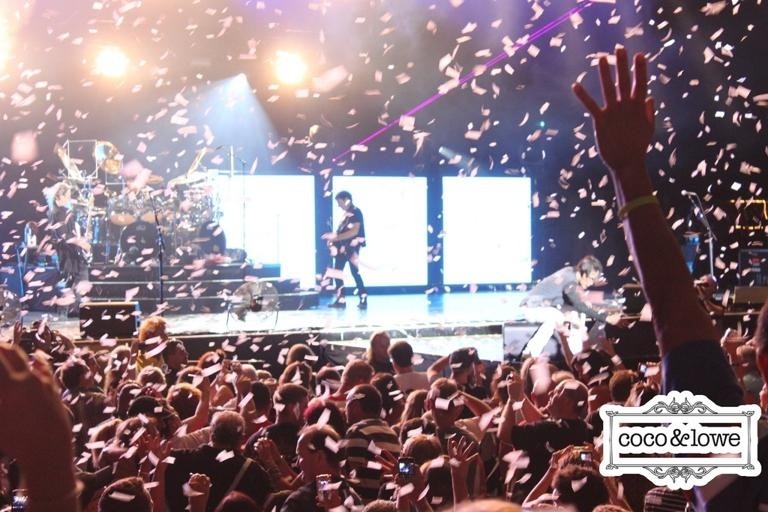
[358,301,367,309]
[329,301,346,308]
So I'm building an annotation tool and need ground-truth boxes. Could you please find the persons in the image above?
[514,257,620,361]
[326,188,368,313]
[1,270,760,510]
[47,183,92,254]
[569,40,762,508]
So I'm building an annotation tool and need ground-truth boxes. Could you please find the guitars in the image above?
[326,217,349,248]
[76,192,93,266]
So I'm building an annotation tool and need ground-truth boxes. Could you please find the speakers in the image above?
[79,301,142,340]
[622,284,649,316]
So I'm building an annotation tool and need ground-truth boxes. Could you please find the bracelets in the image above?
[612,195,659,218]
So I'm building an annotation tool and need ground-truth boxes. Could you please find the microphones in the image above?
[141,188,155,192]
[216,144,224,150]
[241,158,247,166]
[681,190,697,197]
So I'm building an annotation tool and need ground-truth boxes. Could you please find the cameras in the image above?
[397,457,417,480]
[226,361,241,372]
[638,362,647,373]
[580,451,593,463]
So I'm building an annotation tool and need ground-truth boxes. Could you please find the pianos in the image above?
[606,283,661,366]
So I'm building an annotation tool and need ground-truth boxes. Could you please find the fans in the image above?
[229,279,282,328]
[0,288,23,336]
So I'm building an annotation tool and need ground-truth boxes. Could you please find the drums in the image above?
[176,223,225,266]
[119,220,160,261]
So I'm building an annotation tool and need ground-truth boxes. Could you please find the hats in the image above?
[128,397,175,420]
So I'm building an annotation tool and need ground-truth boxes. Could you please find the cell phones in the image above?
[316,473,333,505]
[11,488,31,512]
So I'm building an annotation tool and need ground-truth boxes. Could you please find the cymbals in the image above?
[93,141,123,174]
[187,148,205,174]
[169,171,207,186]
